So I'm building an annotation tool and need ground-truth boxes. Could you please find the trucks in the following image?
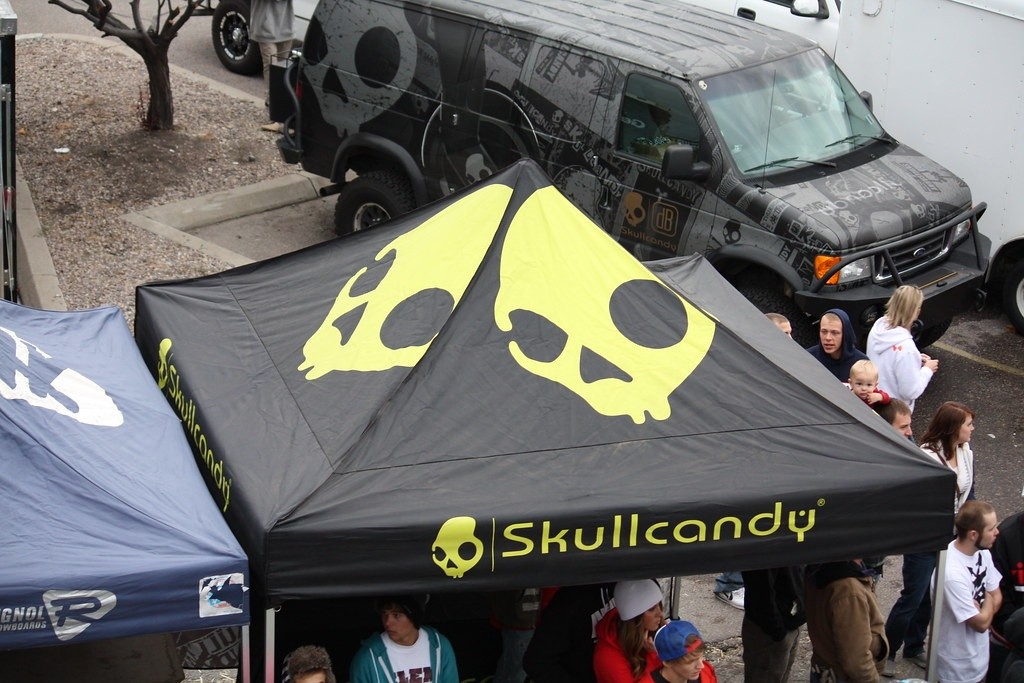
[832,0,1024,345]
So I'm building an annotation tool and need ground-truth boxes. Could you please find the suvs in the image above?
[264,0,994,352]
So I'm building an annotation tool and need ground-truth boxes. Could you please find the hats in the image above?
[614,579,663,620]
[654,621,702,660]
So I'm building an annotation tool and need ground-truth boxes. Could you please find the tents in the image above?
[1,297,255,683]
[133,154,956,683]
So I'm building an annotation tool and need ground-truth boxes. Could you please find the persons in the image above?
[287,644,336,683]
[739,558,928,683]
[930,500,1024,683]
[350,593,460,683]
[712,310,912,609]
[248,0,296,134]
[520,578,717,683]
[880,400,977,677]
[864,285,939,445]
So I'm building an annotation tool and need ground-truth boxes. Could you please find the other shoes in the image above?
[261,122,283,133]
[906,651,928,668]
[881,660,895,676]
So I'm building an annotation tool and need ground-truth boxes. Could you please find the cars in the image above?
[681,0,843,60]
[192,0,318,78]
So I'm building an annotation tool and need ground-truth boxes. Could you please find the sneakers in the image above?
[715,587,745,609]
